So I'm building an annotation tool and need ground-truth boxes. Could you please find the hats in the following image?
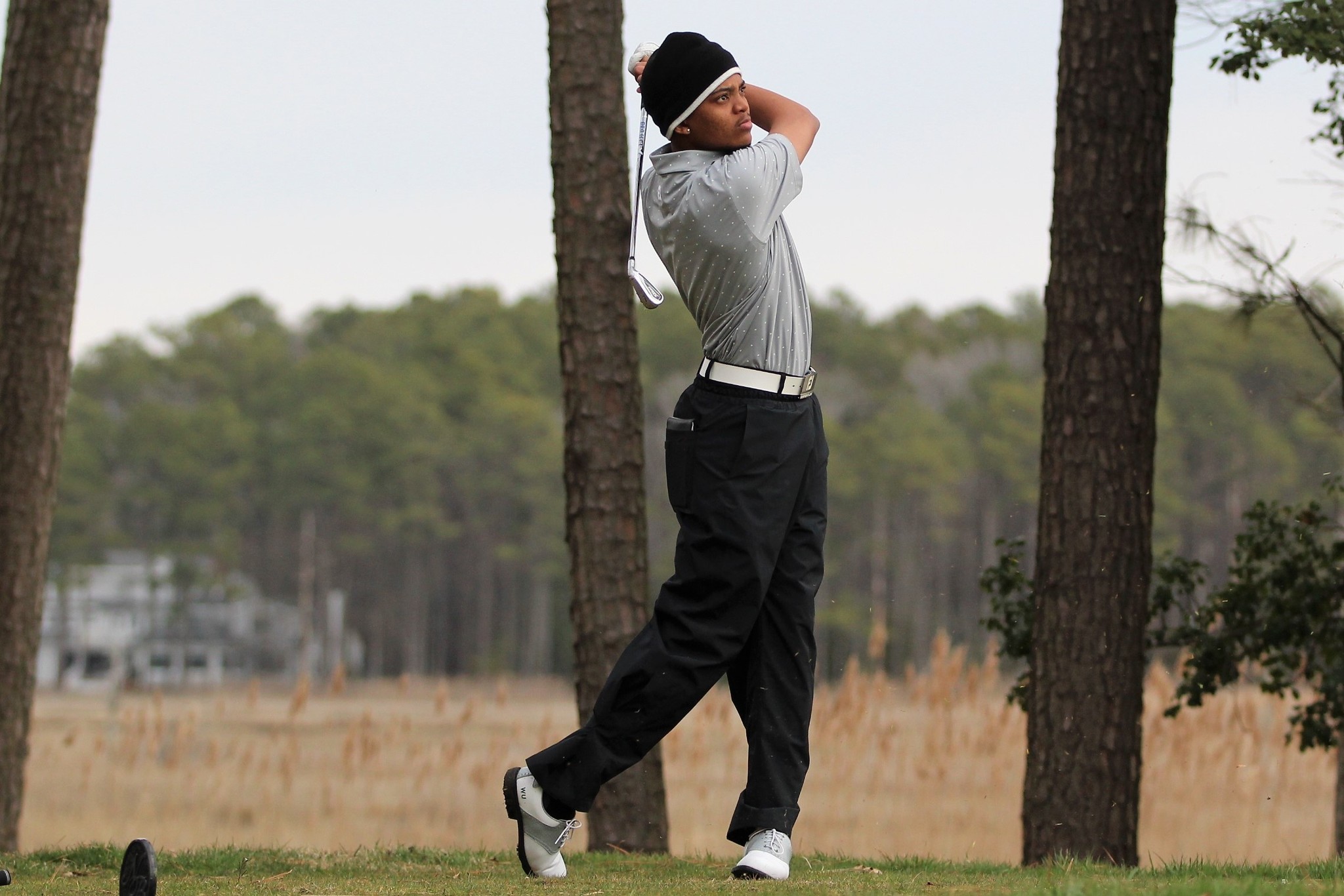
[640,32,742,141]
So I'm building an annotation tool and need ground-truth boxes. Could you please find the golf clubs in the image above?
[627,92,665,311]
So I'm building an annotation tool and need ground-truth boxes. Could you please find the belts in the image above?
[700,357,818,399]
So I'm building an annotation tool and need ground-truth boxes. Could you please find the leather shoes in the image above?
[503,766,582,879]
[732,829,793,879]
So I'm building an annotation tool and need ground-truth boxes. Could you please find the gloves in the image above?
[628,42,660,76]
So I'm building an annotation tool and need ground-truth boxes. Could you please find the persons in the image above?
[502,32,829,879]
